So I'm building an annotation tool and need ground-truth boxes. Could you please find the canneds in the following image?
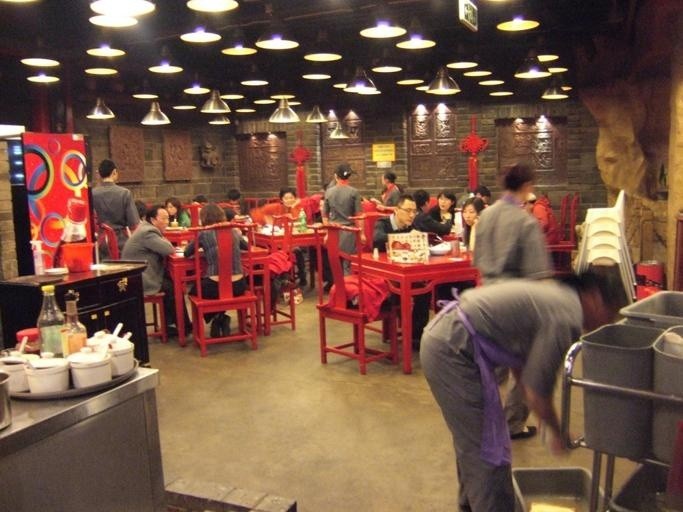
[16,328,40,355]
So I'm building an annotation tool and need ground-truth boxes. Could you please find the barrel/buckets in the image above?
[61,241,95,274]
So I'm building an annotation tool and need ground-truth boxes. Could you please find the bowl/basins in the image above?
[66,347,112,389]
[86,332,114,349]
[0,350,41,394]
[91,336,135,376]
[430,247,450,255]
[24,350,69,393]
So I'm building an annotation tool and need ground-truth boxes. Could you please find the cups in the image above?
[0,373,12,430]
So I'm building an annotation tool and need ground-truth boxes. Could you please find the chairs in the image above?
[574,189,637,304]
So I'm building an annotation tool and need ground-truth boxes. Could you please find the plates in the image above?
[43,268,69,281]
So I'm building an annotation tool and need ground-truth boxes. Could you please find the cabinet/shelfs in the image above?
[0,258,152,369]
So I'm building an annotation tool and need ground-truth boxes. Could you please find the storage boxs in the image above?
[508,462,620,511]
[606,457,678,511]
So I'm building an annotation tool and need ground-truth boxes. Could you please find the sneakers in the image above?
[210,319,220,337]
[221,316,230,337]
[510,426,535,439]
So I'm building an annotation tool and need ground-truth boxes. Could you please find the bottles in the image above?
[451,239,460,257]
[14,327,42,358]
[52,199,88,268]
[298,207,307,232]
[36,284,65,358]
[59,291,87,358]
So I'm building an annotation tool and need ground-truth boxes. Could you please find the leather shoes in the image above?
[167,322,192,335]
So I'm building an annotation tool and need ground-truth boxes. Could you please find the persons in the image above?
[262,188,307,289]
[468,160,556,440]
[134,200,145,219]
[320,163,367,274]
[412,190,452,235]
[460,198,484,239]
[118,205,192,336]
[88,158,139,257]
[417,262,626,510]
[521,192,563,244]
[182,204,248,337]
[371,193,431,352]
[429,191,457,222]
[163,199,190,228]
[191,194,208,209]
[378,172,402,206]
[227,191,241,207]
[472,186,491,208]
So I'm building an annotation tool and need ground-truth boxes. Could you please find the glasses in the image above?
[400,208,419,215]
